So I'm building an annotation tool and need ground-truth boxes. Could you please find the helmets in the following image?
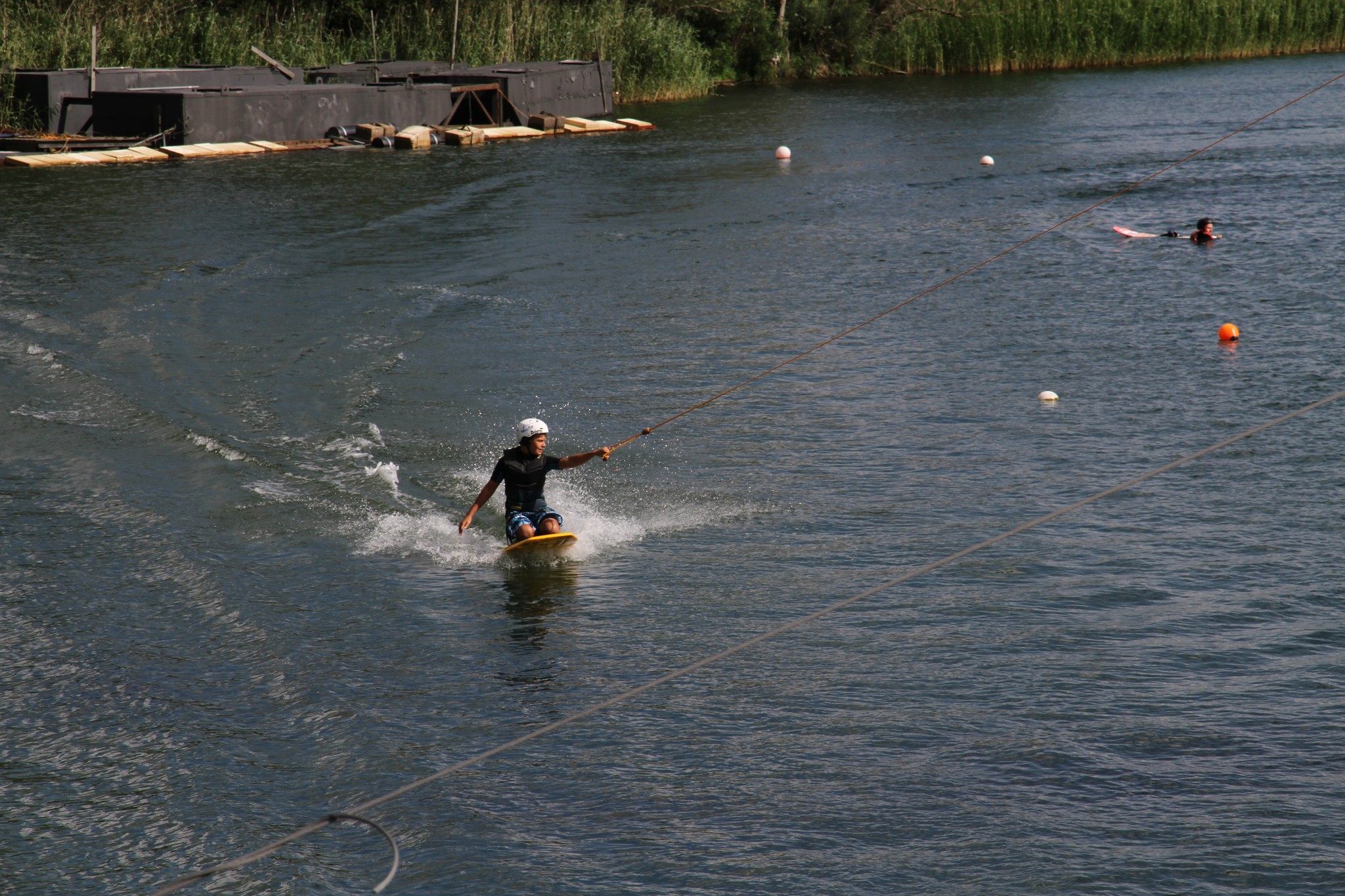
[518,418,549,443]
[1196,218,1213,230]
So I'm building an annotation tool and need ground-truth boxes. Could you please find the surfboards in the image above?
[504,532,578,553]
[1112,226,1224,240]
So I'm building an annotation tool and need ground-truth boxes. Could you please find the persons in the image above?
[458,417,611,542]
[1197,217,1222,238]
